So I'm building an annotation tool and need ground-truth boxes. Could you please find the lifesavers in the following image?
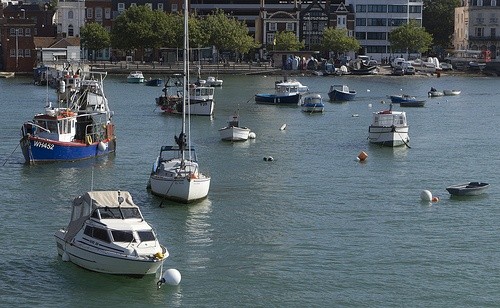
[382,110,392,114]
[65,74,79,78]
[85,135,93,145]
[162,101,176,111]
[62,111,74,114]
[107,123,112,141]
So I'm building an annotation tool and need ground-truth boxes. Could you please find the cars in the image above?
[311,55,487,76]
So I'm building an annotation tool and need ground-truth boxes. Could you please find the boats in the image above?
[399,96,427,108]
[445,181,491,197]
[218,104,251,143]
[390,93,417,104]
[298,88,325,113]
[18,56,118,165]
[255,75,311,106]
[444,88,462,96]
[126,70,145,84]
[144,76,163,87]
[367,102,411,147]
[53,164,170,278]
[428,90,445,97]
[327,83,357,102]
[154,72,223,117]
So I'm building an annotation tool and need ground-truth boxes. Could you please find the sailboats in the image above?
[146,0,212,205]
